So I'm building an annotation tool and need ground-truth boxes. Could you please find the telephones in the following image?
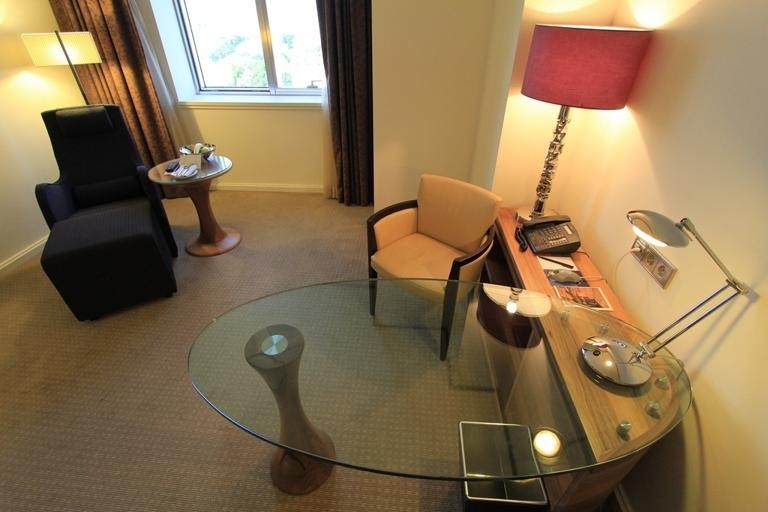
[522,215,580,254]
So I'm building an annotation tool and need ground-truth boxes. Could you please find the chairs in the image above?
[367,174,504,361]
[35,105,178,321]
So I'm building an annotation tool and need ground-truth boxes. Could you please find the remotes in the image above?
[166,162,179,172]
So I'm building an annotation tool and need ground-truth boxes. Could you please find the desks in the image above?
[459,209,679,512]
[188,277,691,494]
[148,156,242,257]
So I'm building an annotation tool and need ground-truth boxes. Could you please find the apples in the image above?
[200,146,209,153]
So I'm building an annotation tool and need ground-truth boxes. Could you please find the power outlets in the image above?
[631,236,677,289]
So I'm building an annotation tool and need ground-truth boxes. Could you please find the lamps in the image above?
[580,211,748,386]
[21,30,103,105]
[514,24,654,223]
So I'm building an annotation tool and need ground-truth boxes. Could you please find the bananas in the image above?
[190,142,203,155]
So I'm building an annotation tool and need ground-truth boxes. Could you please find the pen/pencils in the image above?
[538,255,574,269]
